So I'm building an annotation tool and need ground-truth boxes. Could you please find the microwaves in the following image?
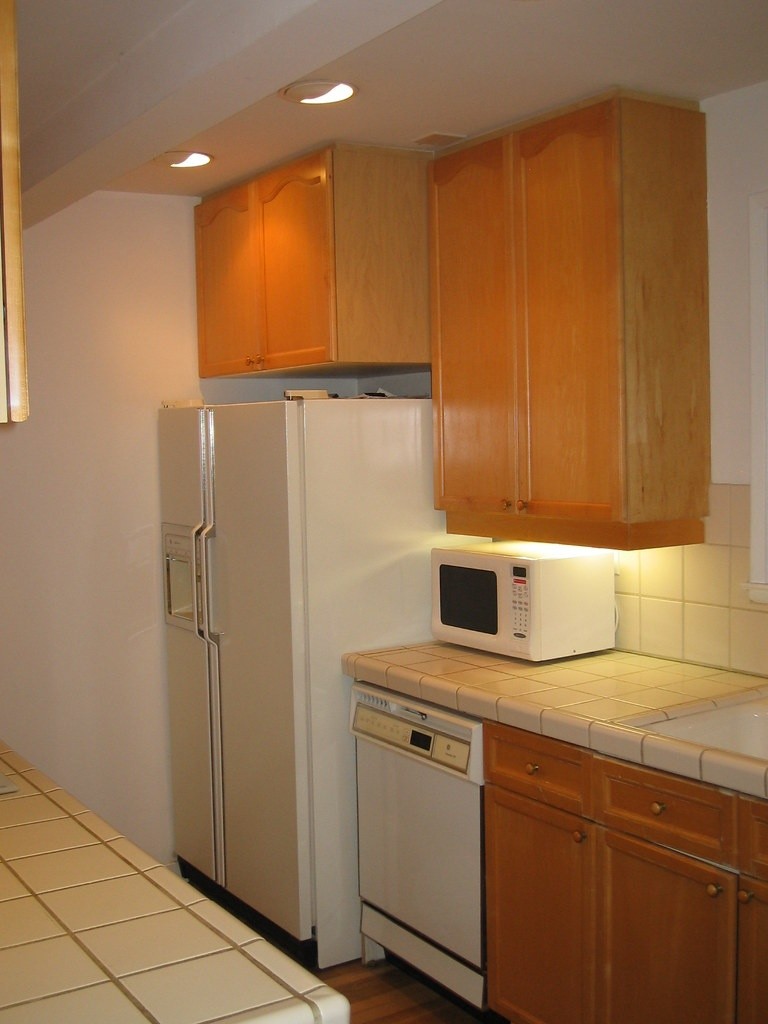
[431,540,617,661]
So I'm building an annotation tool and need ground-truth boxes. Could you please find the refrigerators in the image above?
[156,397,447,971]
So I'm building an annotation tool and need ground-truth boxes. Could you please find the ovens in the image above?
[348,683,485,1011]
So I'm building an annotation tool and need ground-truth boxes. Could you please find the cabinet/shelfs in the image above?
[193,92,713,553]
[481,722,768,1024]
